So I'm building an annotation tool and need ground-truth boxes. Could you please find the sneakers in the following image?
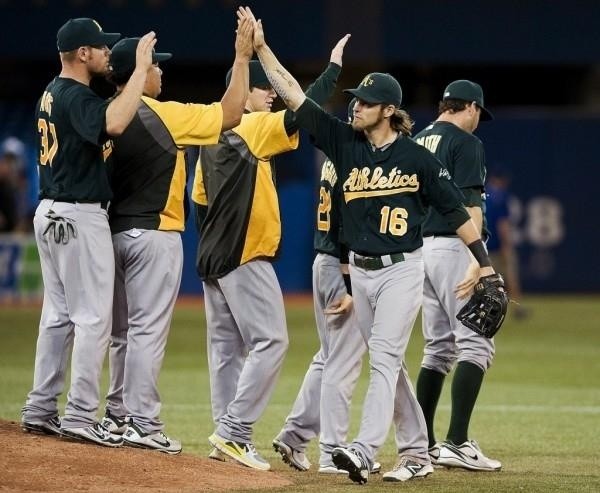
[332,446,371,485]
[383,453,436,482]
[123,424,182,452]
[320,452,383,474]
[59,420,124,447]
[210,445,239,463]
[442,443,502,473]
[425,444,444,464]
[101,416,127,435]
[273,436,314,472]
[23,416,65,434]
[211,430,272,471]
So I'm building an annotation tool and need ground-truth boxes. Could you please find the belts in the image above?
[345,252,404,271]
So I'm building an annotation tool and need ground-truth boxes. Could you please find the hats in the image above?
[108,39,172,71]
[344,73,403,108]
[57,19,119,51]
[444,80,494,117]
[226,61,277,90]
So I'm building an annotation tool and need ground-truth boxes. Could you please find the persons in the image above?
[103,35,251,453]
[191,32,351,472]
[273,152,383,472]
[237,4,496,483]
[22,16,156,447]
[483,172,513,295]
[412,78,502,473]
